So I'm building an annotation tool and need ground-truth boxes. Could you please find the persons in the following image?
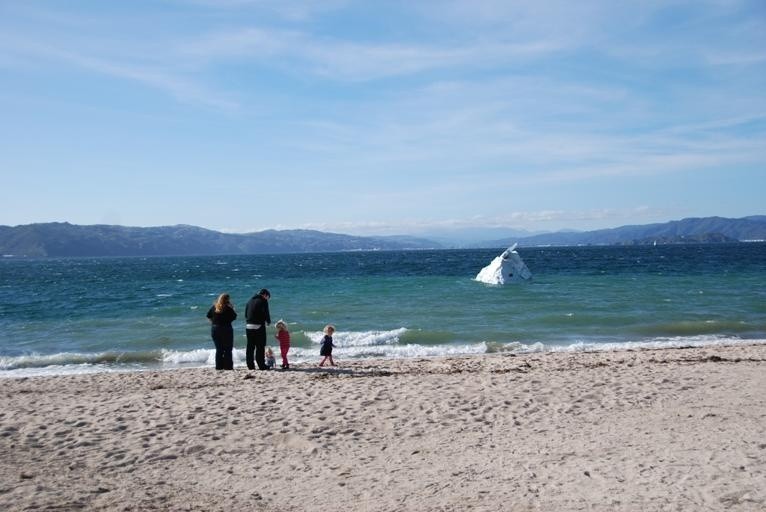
[273,319,291,370]
[265,345,277,370]
[244,289,271,370]
[320,324,337,366]
[207,293,237,370]
